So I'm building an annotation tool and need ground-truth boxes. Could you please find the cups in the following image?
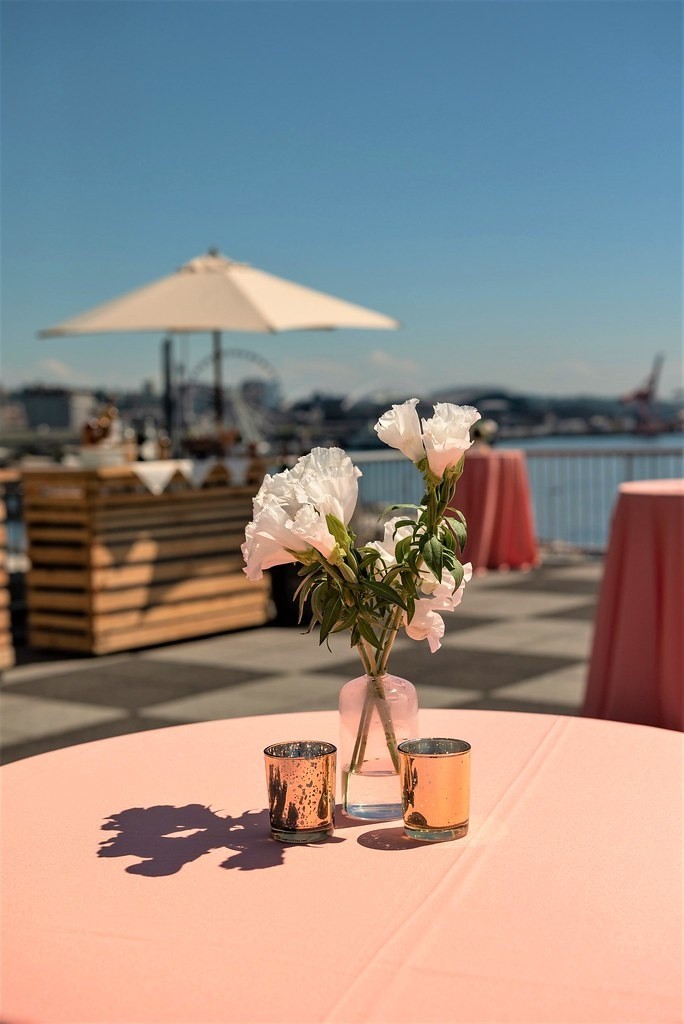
[262,742,337,845]
[395,736,471,842]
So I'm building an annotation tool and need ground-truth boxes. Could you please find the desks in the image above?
[20,460,271,655]
[0,709,684,1024]
[443,449,541,576]
[580,478,684,736]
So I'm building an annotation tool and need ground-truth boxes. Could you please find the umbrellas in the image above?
[35,250,400,424]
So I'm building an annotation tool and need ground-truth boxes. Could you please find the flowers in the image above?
[240,398,482,813]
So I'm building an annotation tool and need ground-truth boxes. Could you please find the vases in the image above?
[334,674,419,822]
